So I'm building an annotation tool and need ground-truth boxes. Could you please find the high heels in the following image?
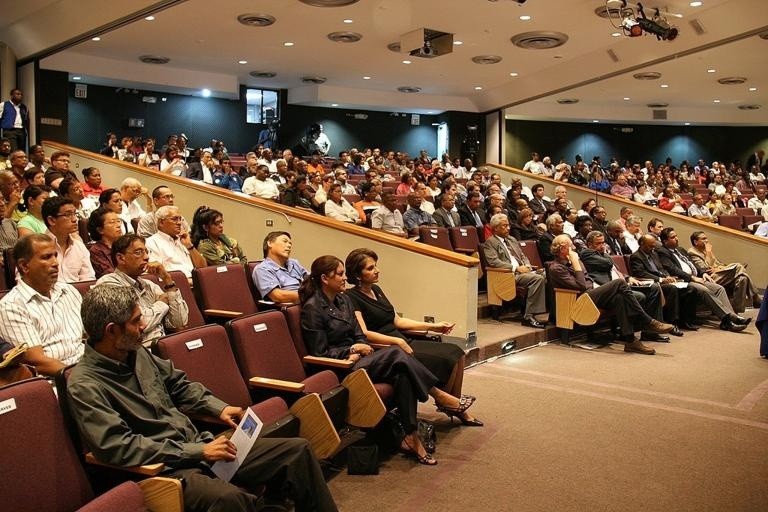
[400,439,436,465]
[434,393,482,426]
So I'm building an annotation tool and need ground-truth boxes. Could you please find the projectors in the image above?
[408,45,438,59]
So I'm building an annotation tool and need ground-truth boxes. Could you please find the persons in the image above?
[1,140,483,511]
[1,89,26,152]
[104,125,768,354]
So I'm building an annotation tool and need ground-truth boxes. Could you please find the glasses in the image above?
[57,212,78,218]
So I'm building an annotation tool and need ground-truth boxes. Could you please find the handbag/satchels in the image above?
[417,421,436,453]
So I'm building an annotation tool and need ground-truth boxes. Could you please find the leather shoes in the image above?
[678,320,697,330]
[521,317,545,328]
[719,316,752,331]
[641,319,683,341]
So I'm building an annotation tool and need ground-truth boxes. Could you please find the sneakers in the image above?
[624,338,655,355]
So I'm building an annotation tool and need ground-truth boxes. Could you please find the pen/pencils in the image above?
[441,323,456,336]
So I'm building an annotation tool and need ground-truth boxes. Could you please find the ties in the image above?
[614,239,622,254]
[504,239,522,266]
[673,249,695,276]
[473,210,481,226]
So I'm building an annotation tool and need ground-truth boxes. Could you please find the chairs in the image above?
[439,151,768,343]
[0,147,438,511]
